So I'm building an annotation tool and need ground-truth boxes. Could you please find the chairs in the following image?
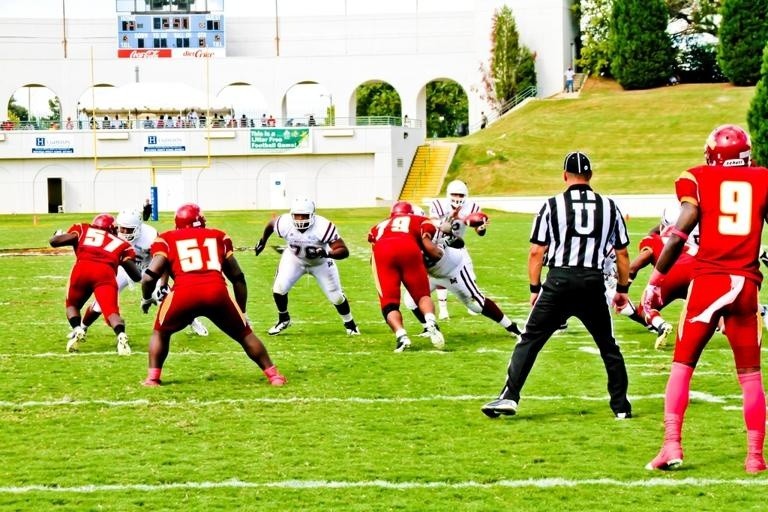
[23,121,277,129]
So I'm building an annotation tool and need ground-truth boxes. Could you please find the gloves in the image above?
[641,288,663,313]
[140,298,156,315]
[134,261,143,274]
[255,240,264,256]
[317,248,328,258]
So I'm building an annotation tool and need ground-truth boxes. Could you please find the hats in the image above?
[564,152,590,174]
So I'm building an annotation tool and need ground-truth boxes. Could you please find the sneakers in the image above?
[654,326,674,351]
[191,318,207,336]
[345,323,361,335]
[481,400,517,419]
[117,333,131,355]
[744,458,766,473]
[269,320,289,334]
[139,379,162,386]
[392,312,448,351]
[270,376,287,385]
[645,447,684,470]
[67,326,87,352]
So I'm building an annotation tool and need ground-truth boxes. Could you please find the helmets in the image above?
[92,210,142,242]
[660,203,681,226]
[446,181,468,207]
[175,204,206,227]
[291,196,316,229]
[706,125,751,166]
[391,201,425,218]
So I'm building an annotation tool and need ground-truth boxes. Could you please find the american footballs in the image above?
[463,212,488,228]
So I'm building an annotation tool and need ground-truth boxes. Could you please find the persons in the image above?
[141,203,287,387]
[2,120,14,130]
[210,113,255,127]
[404,115,410,127]
[480,111,488,128]
[550,241,657,335]
[144,109,207,128]
[77,107,132,129]
[479,150,636,419]
[65,117,74,129]
[308,115,315,126]
[564,65,576,93]
[49,198,209,356]
[285,118,302,127]
[629,123,767,475]
[262,114,275,127]
[367,178,520,354]
[24,122,35,130]
[254,198,359,336]
